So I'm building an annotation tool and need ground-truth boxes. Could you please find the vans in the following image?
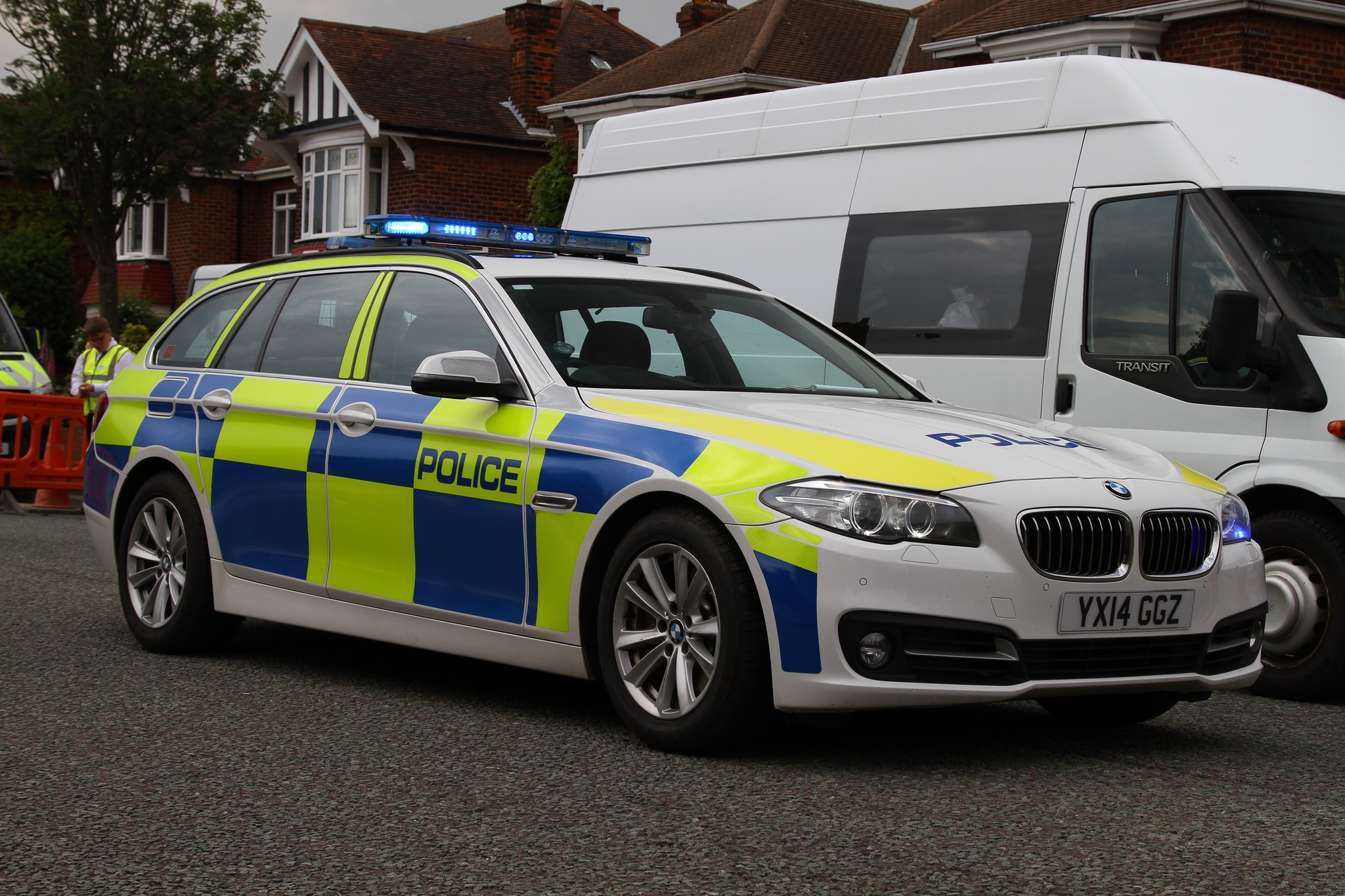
[0,295,55,505]
[556,52,1345,707]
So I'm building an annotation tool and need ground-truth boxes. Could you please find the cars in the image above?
[85,214,1271,752]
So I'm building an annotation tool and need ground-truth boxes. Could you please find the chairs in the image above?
[579,321,651,370]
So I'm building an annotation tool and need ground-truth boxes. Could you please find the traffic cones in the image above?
[23,417,85,516]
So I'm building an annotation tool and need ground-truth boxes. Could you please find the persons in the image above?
[69,318,137,434]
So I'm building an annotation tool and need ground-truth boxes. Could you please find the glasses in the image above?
[89,329,109,342]
